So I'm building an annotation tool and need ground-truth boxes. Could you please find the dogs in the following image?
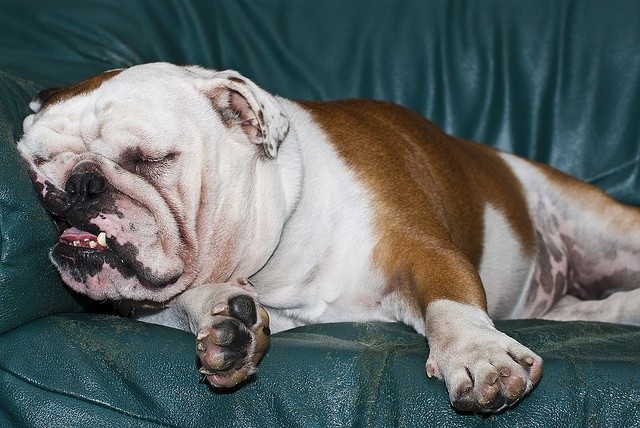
[18,62,640,415]
